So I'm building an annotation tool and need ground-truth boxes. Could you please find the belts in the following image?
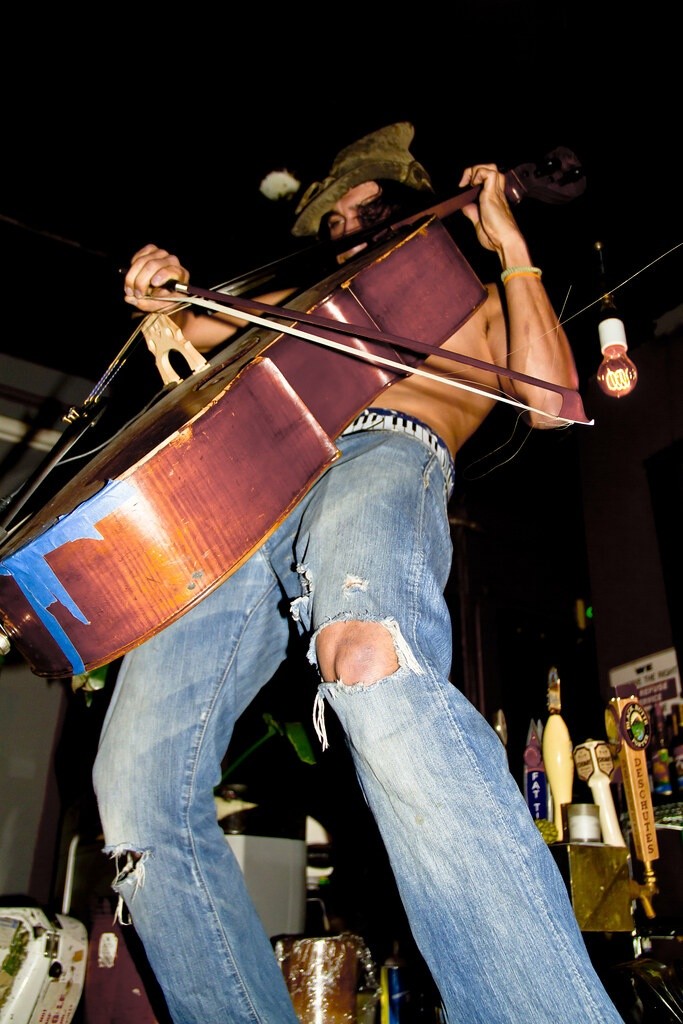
[341,413,456,502]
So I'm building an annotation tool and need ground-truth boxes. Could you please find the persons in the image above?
[91,123,622,1023]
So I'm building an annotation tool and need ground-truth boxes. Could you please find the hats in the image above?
[291,122,439,238]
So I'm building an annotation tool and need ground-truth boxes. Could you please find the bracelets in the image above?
[500,267,543,283]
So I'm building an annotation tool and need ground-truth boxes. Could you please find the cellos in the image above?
[0,143,591,676]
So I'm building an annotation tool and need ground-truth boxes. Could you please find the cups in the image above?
[566,803,600,842]
